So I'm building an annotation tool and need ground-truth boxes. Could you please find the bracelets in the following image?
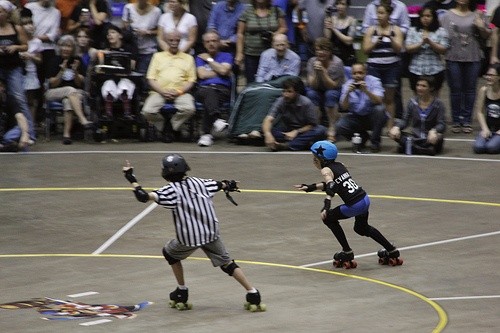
[207,58,214,62]
[178,89,183,94]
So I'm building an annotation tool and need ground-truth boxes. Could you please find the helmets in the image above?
[311,141,337,160]
[163,154,190,173]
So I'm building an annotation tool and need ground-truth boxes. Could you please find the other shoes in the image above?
[160,119,179,143]
[80,121,96,130]
[198,134,212,147]
[371,141,378,152]
[102,113,112,119]
[124,113,133,121]
[272,142,287,151]
[63,135,72,144]
[213,118,227,132]
[251,129,261,138]
[360,134,368,146]
[239,132,249,138]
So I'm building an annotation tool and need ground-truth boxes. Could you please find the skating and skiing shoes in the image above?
[333,251,357,269]
[244,290,266,313]
[169,286,192,310]
[377,248,403,266]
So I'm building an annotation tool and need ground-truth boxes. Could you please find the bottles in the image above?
[330,7,338,28]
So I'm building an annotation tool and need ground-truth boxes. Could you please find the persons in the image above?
[261,76,326,152]
[335,62,386,152]
[0,0,500,149]
[390,76,446,156]
[123,153,264,311]
[473,63,500,154]
[292,140,403,269]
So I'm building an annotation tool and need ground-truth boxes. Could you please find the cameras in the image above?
[353,83,361,89]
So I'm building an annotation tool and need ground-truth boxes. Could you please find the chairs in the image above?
[38,66,237,142]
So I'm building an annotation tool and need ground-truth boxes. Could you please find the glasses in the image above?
[485,73,500,78]
